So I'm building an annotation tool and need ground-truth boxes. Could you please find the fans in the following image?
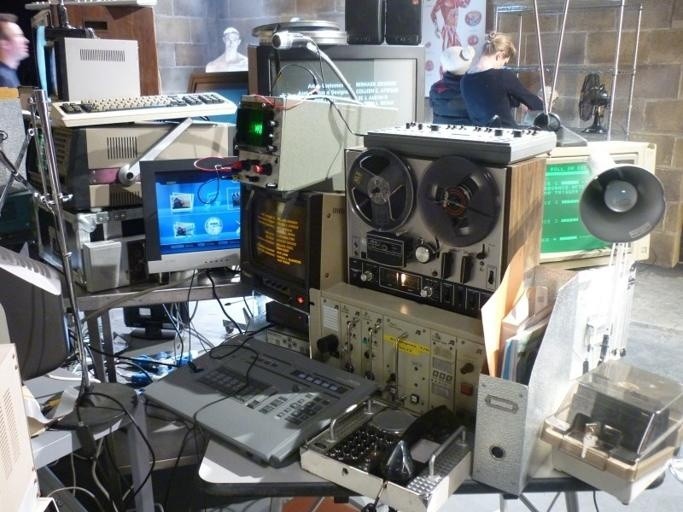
[578,73,611,134]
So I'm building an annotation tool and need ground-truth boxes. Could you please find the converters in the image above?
[72,424,97,456]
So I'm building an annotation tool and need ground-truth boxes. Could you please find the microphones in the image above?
[271,31,311,49]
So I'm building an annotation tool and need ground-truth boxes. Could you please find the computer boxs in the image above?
[54,37,141,102]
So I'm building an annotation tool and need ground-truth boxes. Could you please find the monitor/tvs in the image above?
[138,157,242,288]
[536,141,658,264]
[247,190,346,313]
[0,245,70,381]
[247,43,427,123]
[123,302,190,342]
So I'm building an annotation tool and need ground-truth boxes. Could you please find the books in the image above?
[496,285,550,384]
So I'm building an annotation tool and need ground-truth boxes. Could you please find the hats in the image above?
[440,46,475,76]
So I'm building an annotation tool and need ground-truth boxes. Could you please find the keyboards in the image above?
[52,92,238,128]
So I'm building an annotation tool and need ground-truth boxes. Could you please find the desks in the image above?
[23,366,155,512]
[197,430,666,499]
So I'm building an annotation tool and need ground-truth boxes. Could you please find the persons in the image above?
[0,11,40,88]
[427,43,475,125]
[458,31,559,128]
[204,23,248,74]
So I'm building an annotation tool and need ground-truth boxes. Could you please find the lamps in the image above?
[578,141,666,244]
